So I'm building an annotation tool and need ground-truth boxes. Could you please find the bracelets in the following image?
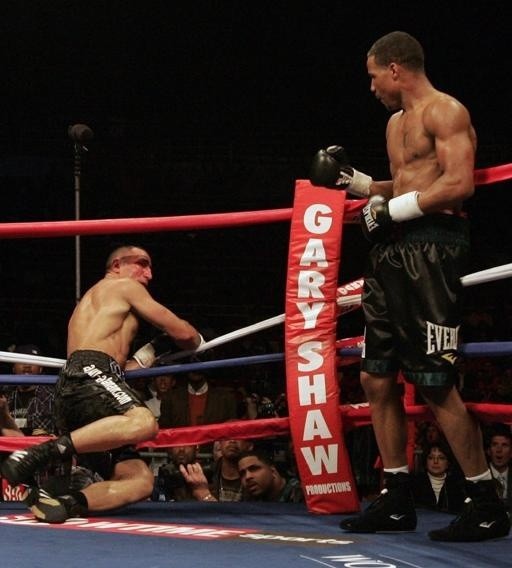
[202,494,213,501]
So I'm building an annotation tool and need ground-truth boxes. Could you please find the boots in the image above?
[27,486,90,523]
[340,471,417,535]
[1,436,72,483]
[427,478,510,542]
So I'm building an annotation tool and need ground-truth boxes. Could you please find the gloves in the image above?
[359,189,424,242]
[132,332,215,377]
[308,145,372,198]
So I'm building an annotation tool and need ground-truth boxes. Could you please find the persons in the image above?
[0,244,205,523]
[308,30,511,542]
[0,308,511,513]
